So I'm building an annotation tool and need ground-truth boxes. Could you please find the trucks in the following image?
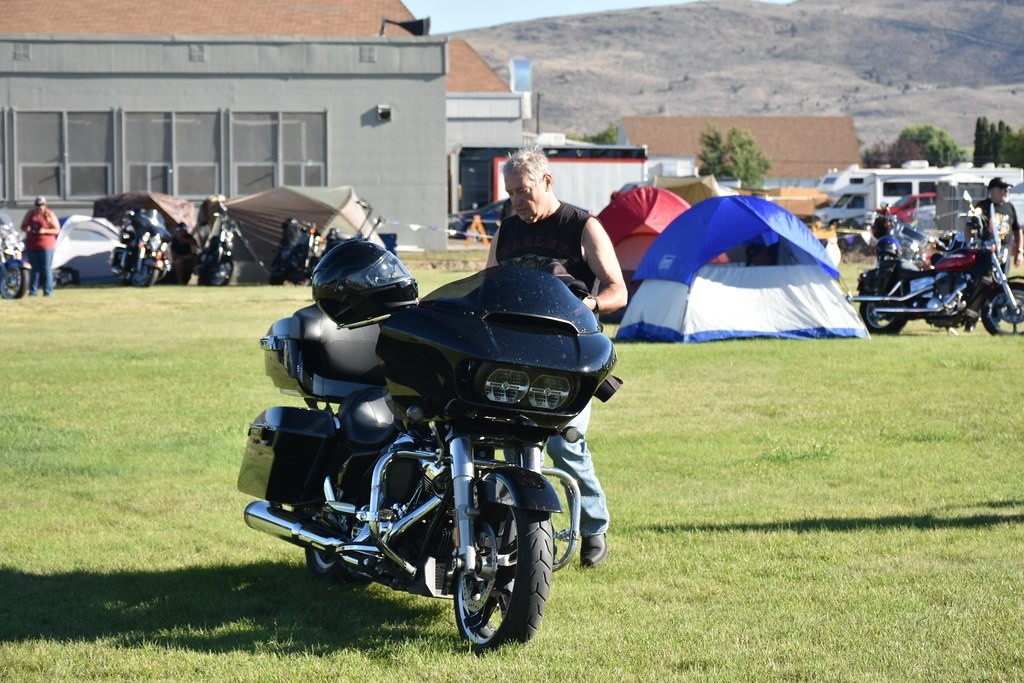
[814,157,1024,286]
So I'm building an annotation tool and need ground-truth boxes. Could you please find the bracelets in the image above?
[1016,249,1023,253]
[588,297,601,313]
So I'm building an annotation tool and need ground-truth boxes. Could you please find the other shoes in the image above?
[965,325,974,332]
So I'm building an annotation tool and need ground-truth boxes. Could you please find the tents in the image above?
[22,185,385,287]
[597,175,719,323]
[616,195,871,339]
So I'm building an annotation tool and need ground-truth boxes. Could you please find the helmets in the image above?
[312,239,419,330]
[875,237,898,262]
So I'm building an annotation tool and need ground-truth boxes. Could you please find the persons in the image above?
[486,149,628,565]
[964,177,1023,333]
[172,223,198,285]
[22,197,60,297]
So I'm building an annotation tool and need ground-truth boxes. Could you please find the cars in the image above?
[447,196,510,243]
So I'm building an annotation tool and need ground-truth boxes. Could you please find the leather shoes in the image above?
[581,533,608,567]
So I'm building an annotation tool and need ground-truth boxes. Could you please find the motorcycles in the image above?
[269,218,365,287]
[236,263,623,661]
[170,202,244,288]
[1,213,33,301]
[849,200,1024,340]
[109,207,187,290]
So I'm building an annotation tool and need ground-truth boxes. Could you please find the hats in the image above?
[988,178,1013,190]
